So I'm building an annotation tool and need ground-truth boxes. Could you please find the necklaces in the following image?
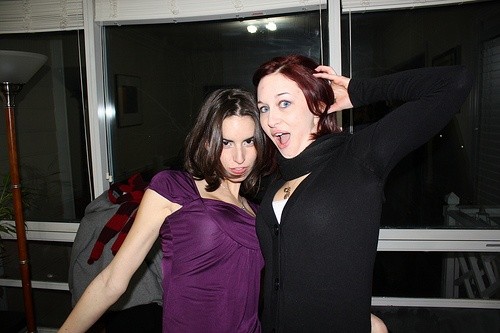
[283,177,302,200]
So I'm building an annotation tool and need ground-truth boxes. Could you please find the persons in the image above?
[250,54,458,333]
[57,88,280,333]
